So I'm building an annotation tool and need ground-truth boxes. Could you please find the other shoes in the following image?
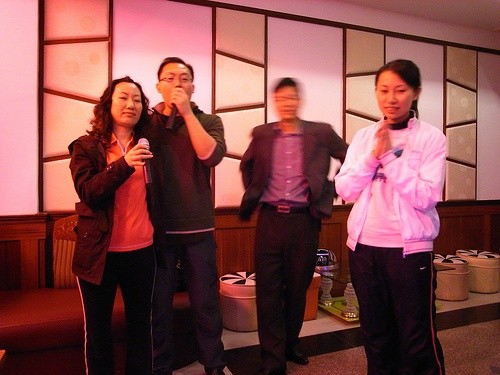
[288,351,309,365]
[204,365,226,375]
[256,368,283,375]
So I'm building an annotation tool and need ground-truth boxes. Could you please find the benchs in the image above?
[0,285,198,375]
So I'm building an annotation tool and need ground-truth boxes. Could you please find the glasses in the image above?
[160,76,193,83]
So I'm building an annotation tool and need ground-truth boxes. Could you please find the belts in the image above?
[264,202,311,214]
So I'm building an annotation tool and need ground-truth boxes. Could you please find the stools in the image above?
[219,271,258,333]
[457,248,500,294]
[433,253,470,301]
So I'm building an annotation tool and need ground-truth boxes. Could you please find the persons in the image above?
[335,59,449,375]
[147,57,226,375]
[240,76,349,375]
[67,76,154,375]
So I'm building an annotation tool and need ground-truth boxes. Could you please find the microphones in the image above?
[138,138,153,187]
[166,104,178,130]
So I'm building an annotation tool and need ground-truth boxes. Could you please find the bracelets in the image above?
[380,150,404,166]
[378,150,393,159]
